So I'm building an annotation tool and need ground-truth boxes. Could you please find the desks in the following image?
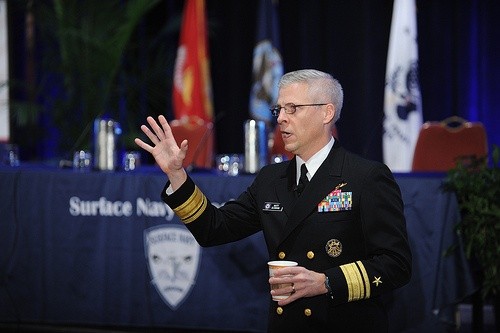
[0,162,451,333]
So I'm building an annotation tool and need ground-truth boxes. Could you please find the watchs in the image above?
[325,273,332,292]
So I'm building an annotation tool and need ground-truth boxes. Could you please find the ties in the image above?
[297,162,309,198]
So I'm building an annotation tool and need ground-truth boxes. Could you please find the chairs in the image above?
[414,122,487,172]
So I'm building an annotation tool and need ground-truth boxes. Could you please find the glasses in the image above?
[269,103,328,117]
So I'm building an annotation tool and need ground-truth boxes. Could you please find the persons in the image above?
[135,70,412,333]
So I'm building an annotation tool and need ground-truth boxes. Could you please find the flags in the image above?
[383,0,421,172]
[173,0,214,168]
[249,0,284,132]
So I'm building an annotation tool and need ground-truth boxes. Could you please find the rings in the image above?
[293,286,295,292]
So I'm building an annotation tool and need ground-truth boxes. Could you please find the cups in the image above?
[245,119,266,174]
[94,119,116,170]
[1,143,20,167]
[73,150,90,168]
[267,261,299,302]
[217,153,239,176]
[125,153,139,171]
